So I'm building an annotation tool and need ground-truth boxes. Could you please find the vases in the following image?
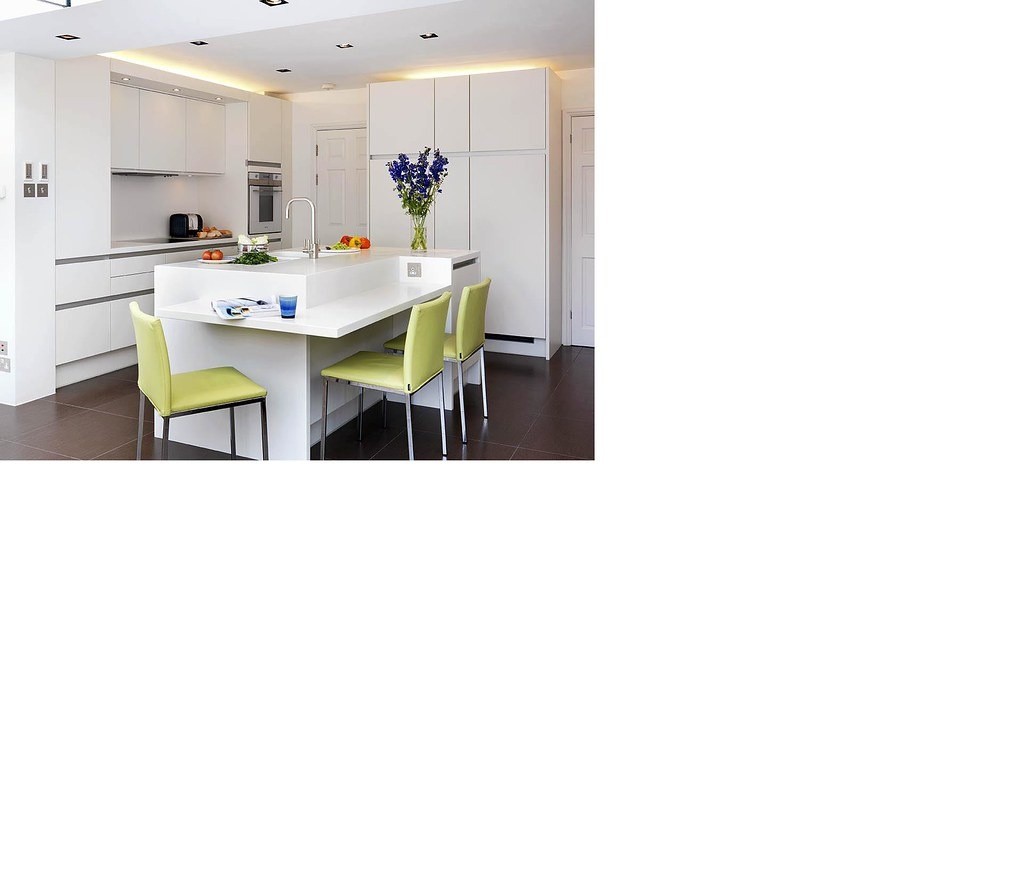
[411,215,429,254]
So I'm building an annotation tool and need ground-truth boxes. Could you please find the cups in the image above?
[279,293,298,319]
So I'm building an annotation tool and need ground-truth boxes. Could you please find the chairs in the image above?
[319,291,453,460]
[128,301,269,461]
[383,276,493,443]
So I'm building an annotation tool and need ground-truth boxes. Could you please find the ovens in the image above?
[249,171,282,236]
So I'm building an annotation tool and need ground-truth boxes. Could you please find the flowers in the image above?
[386,146,450,249]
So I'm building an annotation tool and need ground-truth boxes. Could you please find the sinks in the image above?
[268,251,335,259]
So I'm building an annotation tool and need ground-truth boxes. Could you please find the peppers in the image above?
[341,236,370,249]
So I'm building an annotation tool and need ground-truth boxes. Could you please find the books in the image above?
[209,296,281,320]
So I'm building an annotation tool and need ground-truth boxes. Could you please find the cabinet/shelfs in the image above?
[55,238,282,367]
[366,66,563,361]
[109,84,253,176]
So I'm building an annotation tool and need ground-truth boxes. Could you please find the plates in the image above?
[320,248,360,253]
[196,255,236,263]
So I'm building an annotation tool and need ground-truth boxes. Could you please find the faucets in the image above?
[286,197,319,259]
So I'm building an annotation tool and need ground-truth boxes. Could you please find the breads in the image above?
[197,226,232,238]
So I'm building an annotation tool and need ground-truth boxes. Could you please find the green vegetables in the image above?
[232,251,278,266]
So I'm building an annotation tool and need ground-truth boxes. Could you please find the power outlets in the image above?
[0,358,10,372]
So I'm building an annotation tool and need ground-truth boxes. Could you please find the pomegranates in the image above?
[202,250,223,260]
